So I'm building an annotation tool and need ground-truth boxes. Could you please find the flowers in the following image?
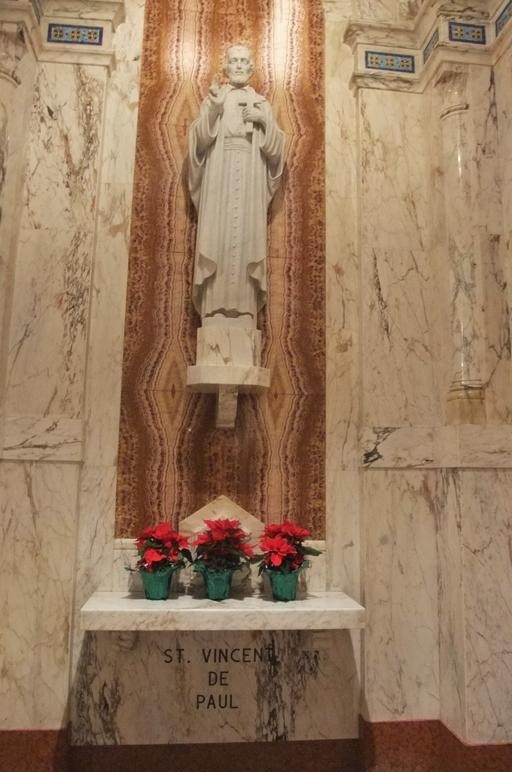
[129,509,324,580]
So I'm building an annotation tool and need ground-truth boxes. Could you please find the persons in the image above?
[188,45,289,364]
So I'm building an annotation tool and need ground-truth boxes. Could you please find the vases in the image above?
[137,565,301,602]
